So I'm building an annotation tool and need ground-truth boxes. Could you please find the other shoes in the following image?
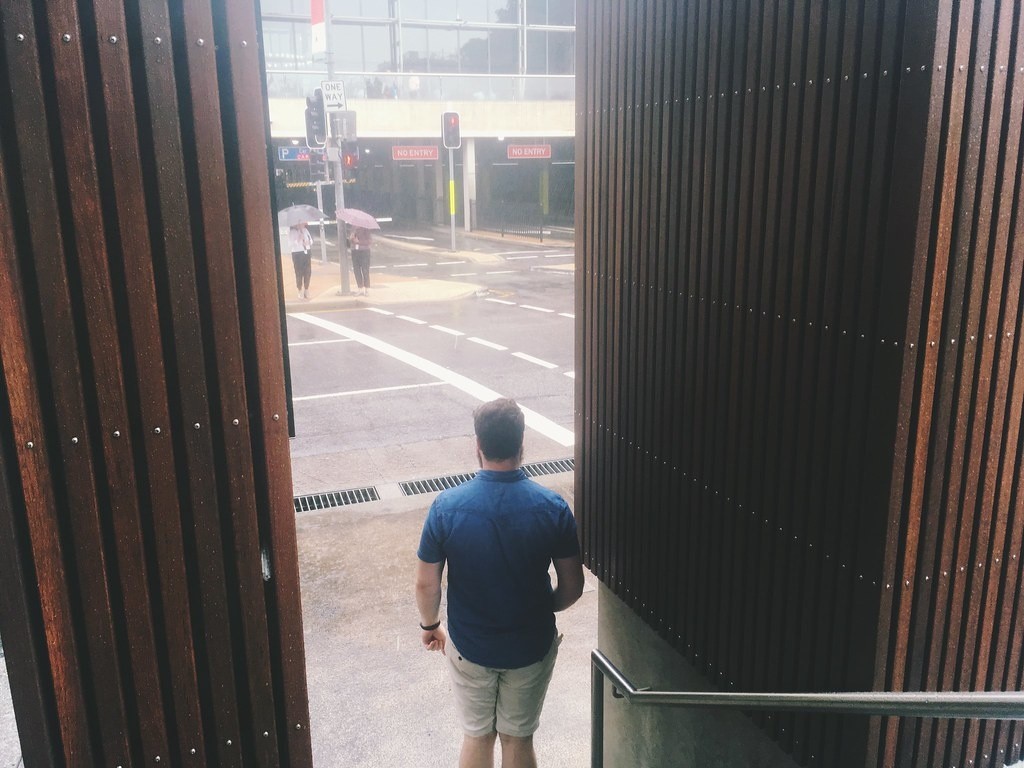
[353,289,371,297]
[297,290,312,300]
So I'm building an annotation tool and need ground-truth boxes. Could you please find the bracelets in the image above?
[419,620,440,631]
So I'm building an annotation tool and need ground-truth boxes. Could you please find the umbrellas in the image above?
[334,208,380,241]
[277,202,330,238]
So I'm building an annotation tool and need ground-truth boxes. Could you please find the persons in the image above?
[287,217,313,301]
[415,398,585,768]
[350,218,372,298]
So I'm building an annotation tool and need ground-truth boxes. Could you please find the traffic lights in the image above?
[442,111,461,149]
[309,151,330,182]
[304,86,329,151]
